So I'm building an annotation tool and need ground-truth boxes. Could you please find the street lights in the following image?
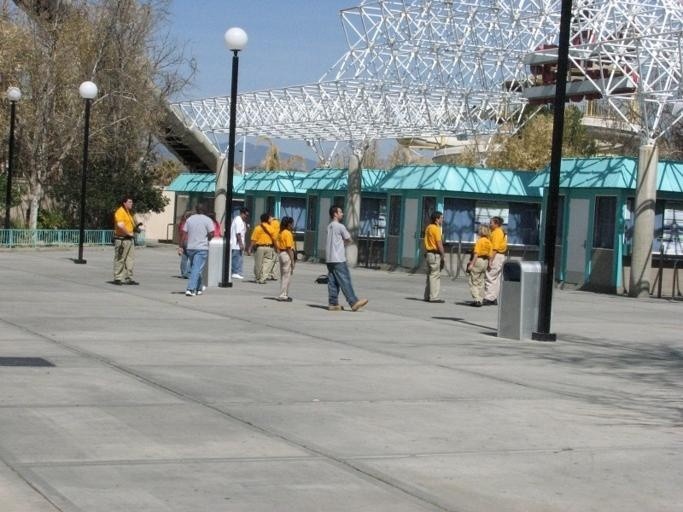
[213,23,248,289]
[70,78,101,265]
[1,84,22,249]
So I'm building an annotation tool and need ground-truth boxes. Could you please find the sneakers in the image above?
[185,285,205,295]
[328,304,343,310]
[351,299,368,311]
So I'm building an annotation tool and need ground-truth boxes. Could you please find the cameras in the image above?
[133,223,143,233]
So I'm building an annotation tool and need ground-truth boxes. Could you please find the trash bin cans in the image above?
[202,236,232,287]
[497,260,542,340]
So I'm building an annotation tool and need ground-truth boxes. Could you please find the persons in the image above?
[175,202,297,302]
[324,205,369,311]
[112,197,143,286]
[466,225,493,308]
[481,216,507,306]
[424,211,446,303]
